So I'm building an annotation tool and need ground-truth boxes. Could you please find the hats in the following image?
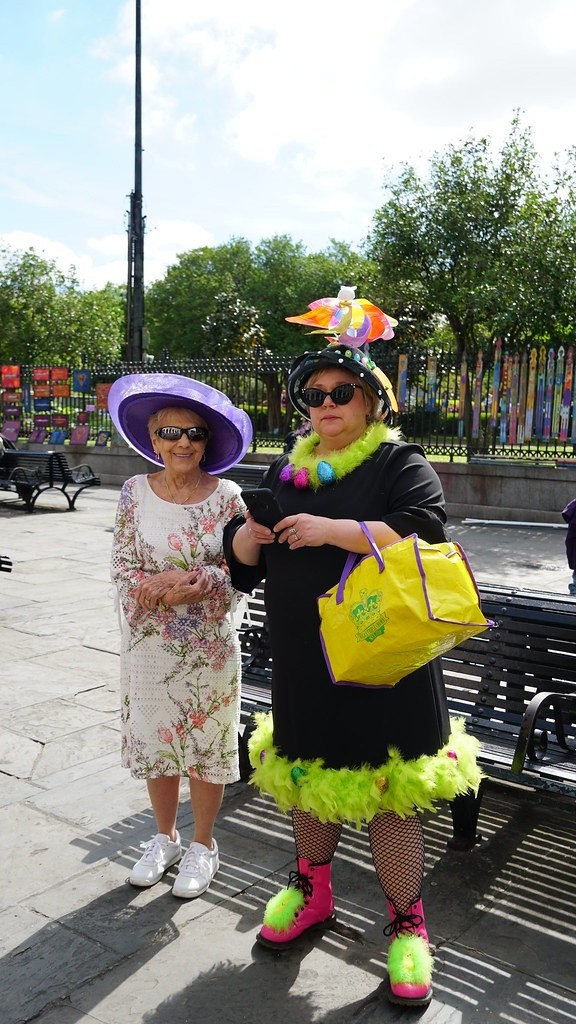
[289,345,394,425]
[107,373,253,475]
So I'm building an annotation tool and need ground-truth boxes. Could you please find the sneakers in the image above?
[171,838,220,898]
[130,830,182,887]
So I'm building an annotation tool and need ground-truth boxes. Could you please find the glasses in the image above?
[301,383,362,408]
[151,426,209,442]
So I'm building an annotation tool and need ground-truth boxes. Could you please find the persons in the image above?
[222,364,485,1004]
[110,408,248,897]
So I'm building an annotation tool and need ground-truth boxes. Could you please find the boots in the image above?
[258,857,338,950]
[385,897,435,1007]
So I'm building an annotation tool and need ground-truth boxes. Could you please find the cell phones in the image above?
[240,487,288,548]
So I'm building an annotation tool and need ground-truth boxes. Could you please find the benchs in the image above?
[213,559,576,851]
[1,449,100,511]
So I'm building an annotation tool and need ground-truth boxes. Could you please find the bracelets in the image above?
[159,597,170,610]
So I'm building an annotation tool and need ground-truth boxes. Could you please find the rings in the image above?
[248,527,251,533]
[204,590,207,595]
[289,527,296,534]
[294,534,299,540]
[145,598,149,603]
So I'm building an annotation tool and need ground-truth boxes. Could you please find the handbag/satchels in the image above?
[317,520,494,688]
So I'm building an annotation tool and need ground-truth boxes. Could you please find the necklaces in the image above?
[164,468,203,503]
